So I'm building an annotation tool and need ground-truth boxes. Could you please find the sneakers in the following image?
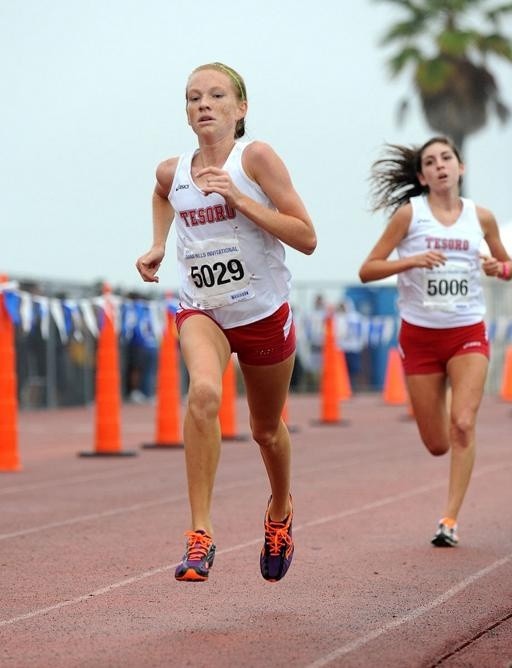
[258,490,296,583]
[175,528,218,583]
[429,517,461,549]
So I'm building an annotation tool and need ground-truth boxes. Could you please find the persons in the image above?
[357,135,512,551]
[133,59,318,591]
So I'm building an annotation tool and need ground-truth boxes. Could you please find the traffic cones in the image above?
[0,274,23,475]
[279,393,298,438]
[400,401,416,421]
[215,350,251,443]
[141,309,189,450]
[501,346,512,403]
[314,314,350,427]
[381,348,402,407]
[77,283,141,459]
[334,348,350,402]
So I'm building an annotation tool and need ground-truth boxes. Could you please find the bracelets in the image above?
[496,255,510,280]
